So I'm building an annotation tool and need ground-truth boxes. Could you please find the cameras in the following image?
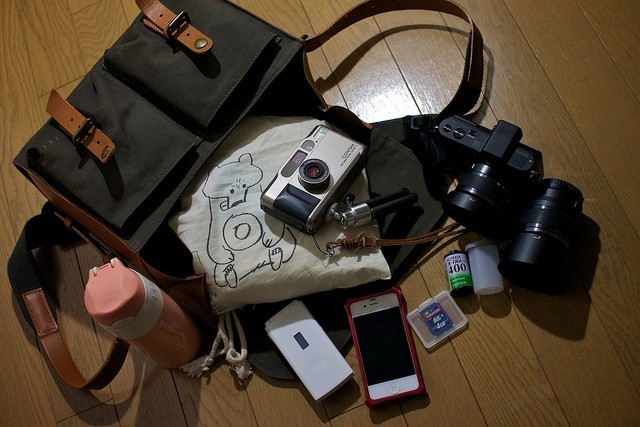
[438,114,535,228]
[259,123,369,234]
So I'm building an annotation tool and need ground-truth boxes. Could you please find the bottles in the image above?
[84,258,201,370]
[465,239,505,296]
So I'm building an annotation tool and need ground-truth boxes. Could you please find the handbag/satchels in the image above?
[7,1,483,392]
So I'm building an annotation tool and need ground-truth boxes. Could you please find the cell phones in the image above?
[345,284,425,407]
